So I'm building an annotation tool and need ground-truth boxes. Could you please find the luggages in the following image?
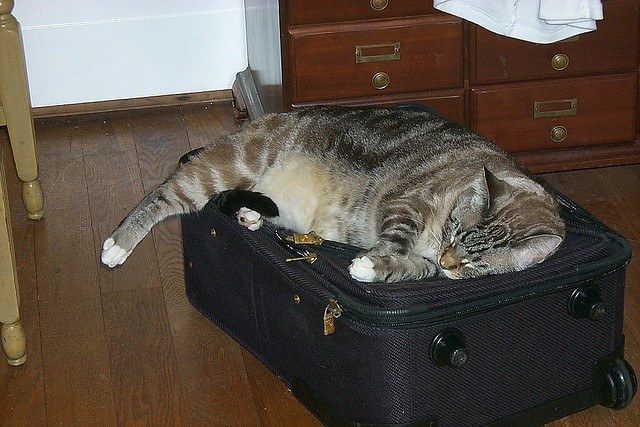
[179,103,638,427]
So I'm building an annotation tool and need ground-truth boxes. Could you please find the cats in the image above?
[101,103,567,283]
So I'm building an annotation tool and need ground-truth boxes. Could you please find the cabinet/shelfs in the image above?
[226,0,640,174]
